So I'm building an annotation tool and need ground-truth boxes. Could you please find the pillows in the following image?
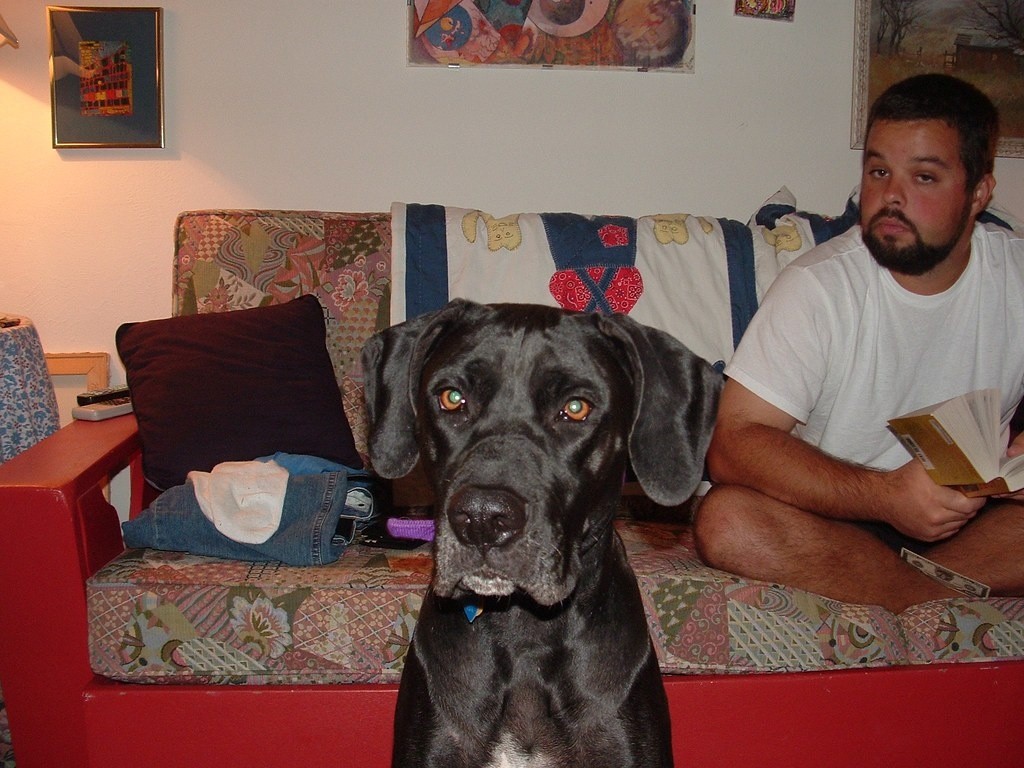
[115,293,365,508]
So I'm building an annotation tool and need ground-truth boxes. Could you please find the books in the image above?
[885,388,1024,497]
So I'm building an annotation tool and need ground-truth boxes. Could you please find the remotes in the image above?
[76,384,130,406]
[72,397,133,420]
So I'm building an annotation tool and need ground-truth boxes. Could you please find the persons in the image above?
[692,74,1024,612]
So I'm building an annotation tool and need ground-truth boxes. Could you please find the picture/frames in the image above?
[45,6,164,150]
[850,0,1023,159]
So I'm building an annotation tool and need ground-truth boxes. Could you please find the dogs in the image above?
[357,296,729,768]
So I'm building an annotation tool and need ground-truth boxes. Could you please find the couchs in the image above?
[0,204,1024,768]
